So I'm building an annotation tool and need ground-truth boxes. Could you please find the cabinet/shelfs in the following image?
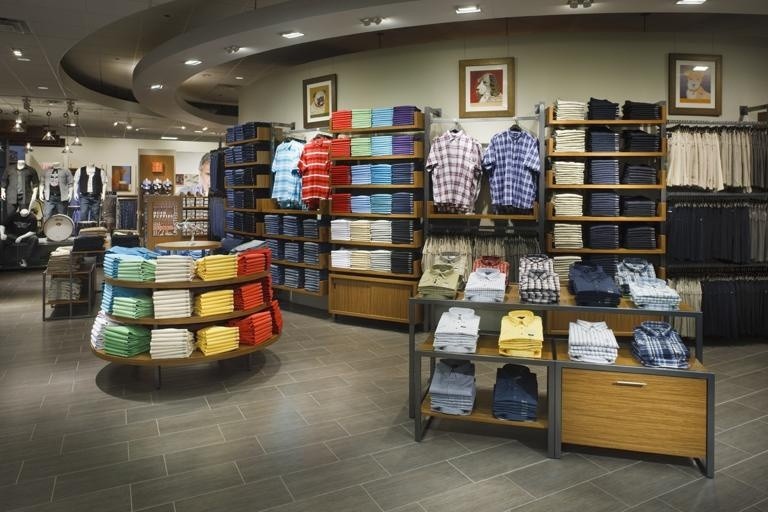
[553,281,716,481]
[408,285,553,458]
[40,234,115,321]
[261,196,325,300]
[544,103,668,257]
[88,236,285,392]
[326,111,425,332]
[221,125,279,237]
[179,191,209,237]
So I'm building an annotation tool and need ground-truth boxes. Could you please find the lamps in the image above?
[1,96,82,155]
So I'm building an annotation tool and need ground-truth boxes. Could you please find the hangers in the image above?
[450,122,466,134]
[665,263,767,282]
[427,228,538,242]
[509,118,521,132]
[665,189,767,209]
[667,119,768,134]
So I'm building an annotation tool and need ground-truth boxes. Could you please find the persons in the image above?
[74,161,109,224]
[195,152,211,198]
[36,159,74,225]
[0,157,40,213]
[0,209,42,273]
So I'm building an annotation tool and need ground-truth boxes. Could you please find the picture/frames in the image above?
[300,72,337,128]
[455,54,516,119]
[666,51,724,118]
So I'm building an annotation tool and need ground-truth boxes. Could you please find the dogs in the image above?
[684,69,711,99]
[310,89,326,115]
[476,73,503,103]
